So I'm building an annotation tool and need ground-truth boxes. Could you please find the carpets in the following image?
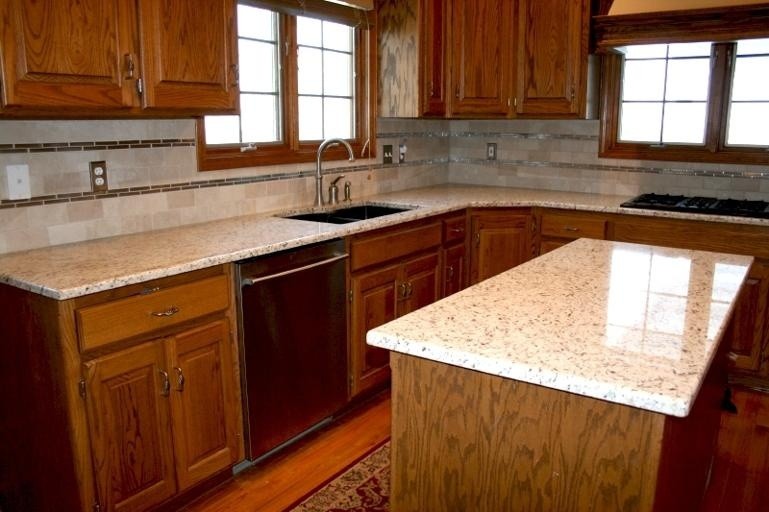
[283,437,391,512]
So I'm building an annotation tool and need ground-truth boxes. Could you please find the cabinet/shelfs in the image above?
[0,263,246,511]
[442,207,468,298]
[0,0,240,116]
[416,0,591,119]
[534,207,610,259]
[468,207,535,287]
[346,214,442,402]
[610,213,768,388]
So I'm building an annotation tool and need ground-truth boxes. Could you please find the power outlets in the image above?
[89,161,108,194]
[487,143,497,160]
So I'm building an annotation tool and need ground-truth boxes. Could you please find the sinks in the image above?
[332,201,420,220]
[276,212,356,225]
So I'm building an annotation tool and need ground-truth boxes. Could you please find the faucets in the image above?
[314,137,355,207]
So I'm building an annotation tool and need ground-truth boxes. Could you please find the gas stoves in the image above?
[620,192,769,221]
